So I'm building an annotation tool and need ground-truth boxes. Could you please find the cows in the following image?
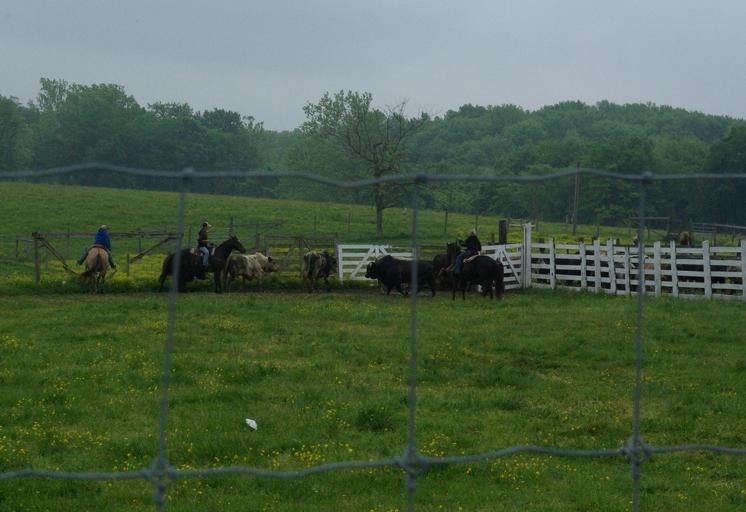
[300,247,338,294]
[364,255,439,298]
[223,252,279,288]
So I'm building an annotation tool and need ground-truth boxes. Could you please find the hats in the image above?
[203,222,212,226]
[100,224,113,230]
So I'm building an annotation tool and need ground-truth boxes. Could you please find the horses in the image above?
[157,234,246,293]
[81,246,109,294]
[445,240,505,301]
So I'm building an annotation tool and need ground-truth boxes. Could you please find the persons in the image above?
[74,224,117,269]
[678,231,690,256]
[197,221,210,272]
[452,228,482,272]
